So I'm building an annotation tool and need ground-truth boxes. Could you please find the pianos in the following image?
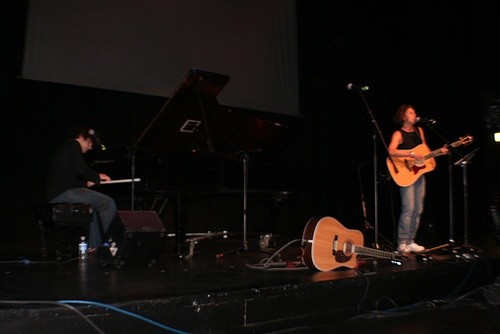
[89,67,306,256]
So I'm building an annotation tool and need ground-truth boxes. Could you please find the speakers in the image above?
[99,210,166,266]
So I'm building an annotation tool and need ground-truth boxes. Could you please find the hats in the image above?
[81,127,103,148]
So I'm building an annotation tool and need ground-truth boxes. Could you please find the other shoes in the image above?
[88,241,118,256]
[397,242,425,252]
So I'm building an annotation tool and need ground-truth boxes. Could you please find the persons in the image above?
[387,105,449,253]
[46,129,116,253]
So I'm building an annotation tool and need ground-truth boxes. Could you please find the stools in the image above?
[48,201,92,259]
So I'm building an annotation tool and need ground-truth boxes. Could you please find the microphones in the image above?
[88,129,104,148]
[347,83,369,91]
[416,117,437,124]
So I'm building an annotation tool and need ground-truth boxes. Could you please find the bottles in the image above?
[79,237,88,262]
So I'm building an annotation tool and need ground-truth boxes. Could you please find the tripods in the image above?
[425,122,481,259]
[215,151,281,260]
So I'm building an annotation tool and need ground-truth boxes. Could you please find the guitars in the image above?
[301,216,411,272]
[385,133,474,187]
